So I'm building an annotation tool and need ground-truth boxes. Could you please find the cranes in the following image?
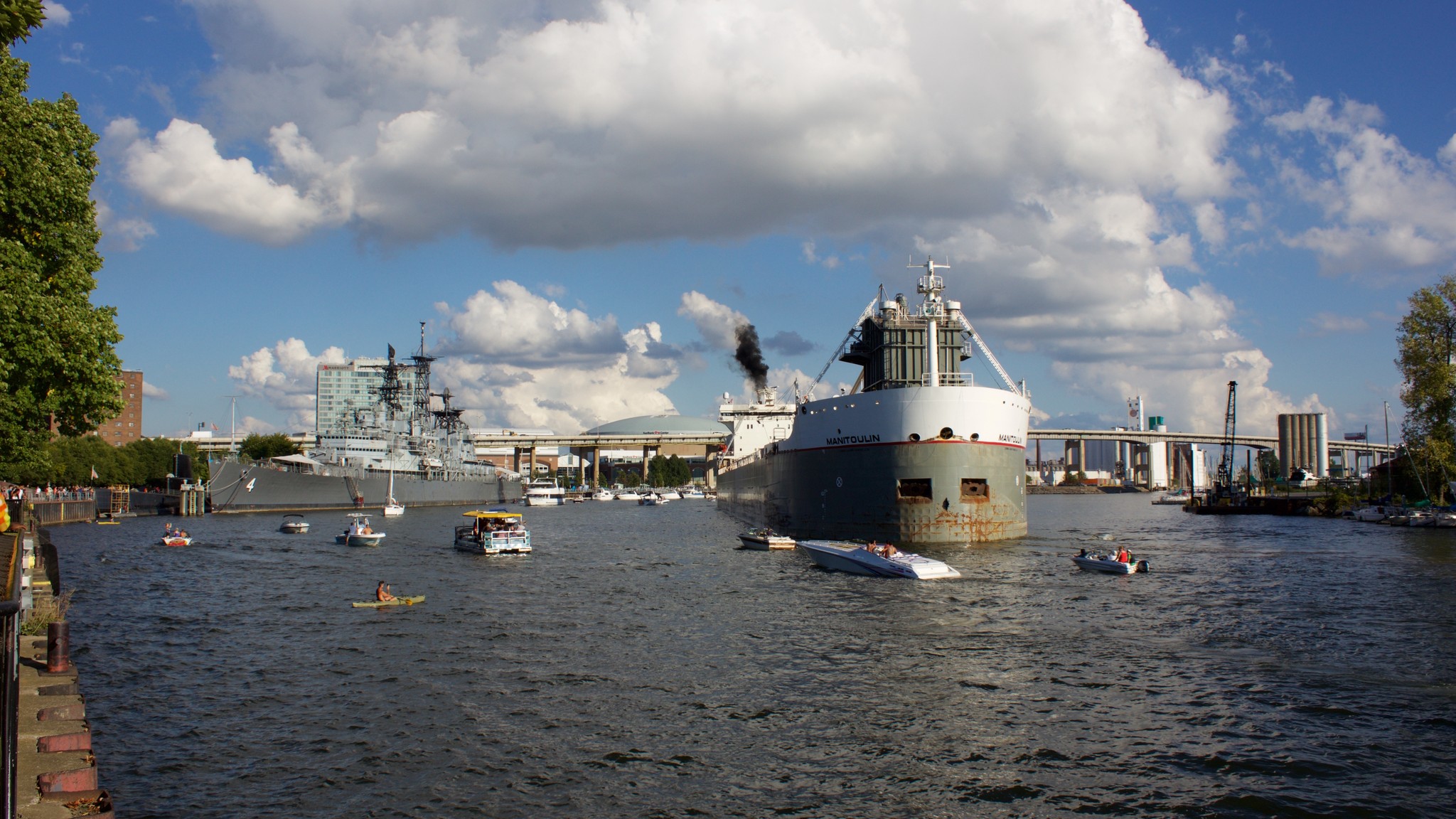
[1214,381,1240,501]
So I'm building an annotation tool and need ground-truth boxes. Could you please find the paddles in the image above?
[396,597,413,605]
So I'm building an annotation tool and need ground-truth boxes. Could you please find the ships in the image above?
[715,253,1033,541]
[207,320,524,514]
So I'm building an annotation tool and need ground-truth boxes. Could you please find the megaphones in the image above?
[924,307,931,315]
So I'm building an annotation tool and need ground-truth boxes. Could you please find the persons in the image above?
[376,581,398,601]
[766,527,772,537]
[0,481,159,533]
[473,518,526,541]
[720,444,727,453]
[1126,549,1136,566]
[865,539,897,559]
[165,523,190,539]
[345,521,373,545]
[1112,546,1129,563]
[1073,548,1094,558]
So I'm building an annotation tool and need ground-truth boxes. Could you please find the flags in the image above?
[211,422,219,431]
[91,467,98,478]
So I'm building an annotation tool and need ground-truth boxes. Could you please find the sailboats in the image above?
[1160,449,1206,502]
[1339,399,1456,533]
[384,468,405,515]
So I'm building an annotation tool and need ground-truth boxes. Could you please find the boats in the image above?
[795,539,961,579]
[454,507,534,555]
[736,528,797,550]
[572,495,585,504]
[162,530,192,547]
[335,513,387,548]
[615,486,717,506]
[1070,550,1151,575]
[522,475,566,506]
[98,522,121,524]
[279,513,310,534]
[352,595,426,609]
[591,487,615,501]
